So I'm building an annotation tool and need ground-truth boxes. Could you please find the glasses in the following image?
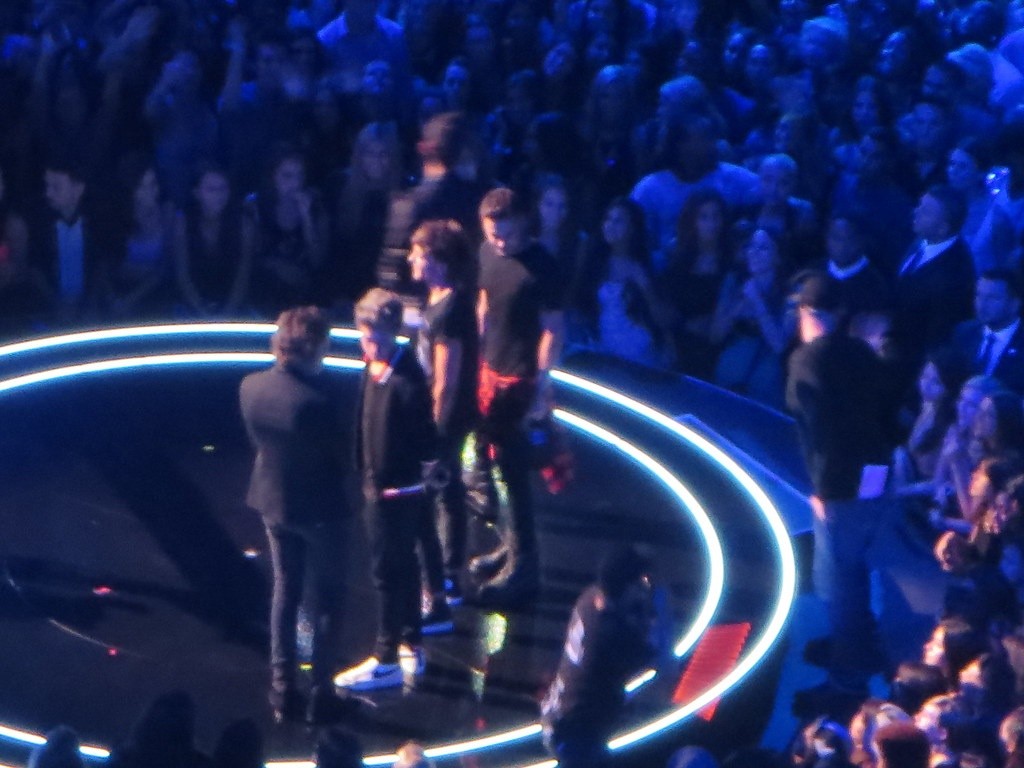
[746,243,771,252]
[746,57,774,69]
[954,394,979,411]
[971,290,1007,306]
[724,44,747,58]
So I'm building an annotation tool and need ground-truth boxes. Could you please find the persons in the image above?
[239,306,357,720]
[0,0,1024,768]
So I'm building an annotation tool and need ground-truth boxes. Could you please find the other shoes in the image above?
[305,693,365,722]
[442,554,464,570]
[419,604,455,637]
[396,642,426,675]
[802,631,885,669]
[475,562,533,607]
[270,689,303,724]
[468,544,509,576]
[332,654,402,693]
[443,575,466,607]
[466,488,499,519]
[793,681,871,720]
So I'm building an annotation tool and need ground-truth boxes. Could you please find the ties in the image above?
[900,246,925,277]
[977,333,995,377]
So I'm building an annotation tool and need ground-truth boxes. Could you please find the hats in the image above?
[803,17,847,45]
[946,40,993,92]
[785,275,841,313]
[415,111,485,157]
